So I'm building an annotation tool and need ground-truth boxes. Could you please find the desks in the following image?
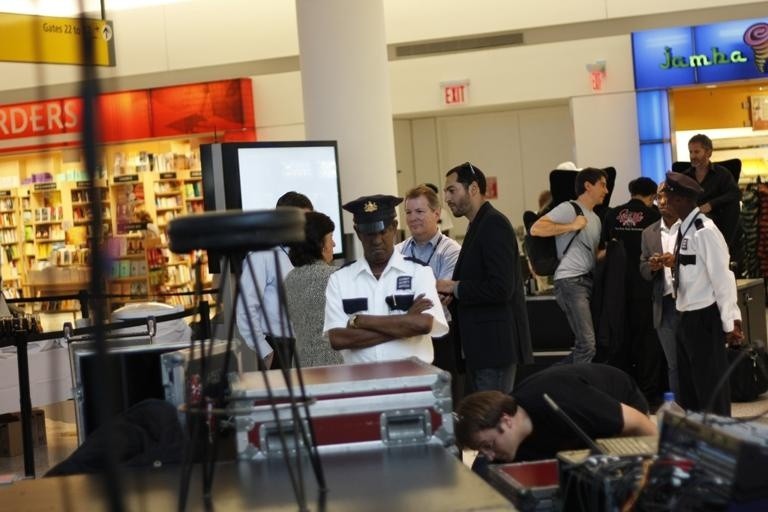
[0,444,522,512]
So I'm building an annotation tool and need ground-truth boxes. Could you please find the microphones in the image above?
[700,338,764,428]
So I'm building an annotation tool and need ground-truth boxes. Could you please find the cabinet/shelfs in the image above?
[735,278,767,352]
[0,138,216,312]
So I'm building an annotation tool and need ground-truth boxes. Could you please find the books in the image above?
[0,151,212,315]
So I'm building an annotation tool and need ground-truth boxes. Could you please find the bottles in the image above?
[656,391,685,434]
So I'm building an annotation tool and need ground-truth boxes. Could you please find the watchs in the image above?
[349,313,360,328]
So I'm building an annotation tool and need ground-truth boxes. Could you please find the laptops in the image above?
[544,393,660,458]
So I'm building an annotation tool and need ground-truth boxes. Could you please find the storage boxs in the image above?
[487,457,563,512]
[227,356,453,456]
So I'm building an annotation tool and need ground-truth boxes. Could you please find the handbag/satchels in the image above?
[728,334,768,403]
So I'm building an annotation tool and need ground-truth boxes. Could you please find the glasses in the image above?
[464,160,477,180]
[473,430,495,460]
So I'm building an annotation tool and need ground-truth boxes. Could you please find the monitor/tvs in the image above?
[201,140,348,275]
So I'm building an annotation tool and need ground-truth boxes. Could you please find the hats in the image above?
[341,194,404,235]
[659,171,704,201]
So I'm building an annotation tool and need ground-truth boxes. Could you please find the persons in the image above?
[530,167,608,366]
[639,181,685,405]
[443,162,536,396]
[393,185,461,370]
[522,190,555,293]
[455,363,658,512]
[236,190,314,372]
[321,194,450,365]
[284,211,340,369]
[664,173,747,418]
[680,134,742,248]
[604,176,660,391]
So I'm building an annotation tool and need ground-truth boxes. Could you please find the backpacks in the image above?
[523,201,588,276]
